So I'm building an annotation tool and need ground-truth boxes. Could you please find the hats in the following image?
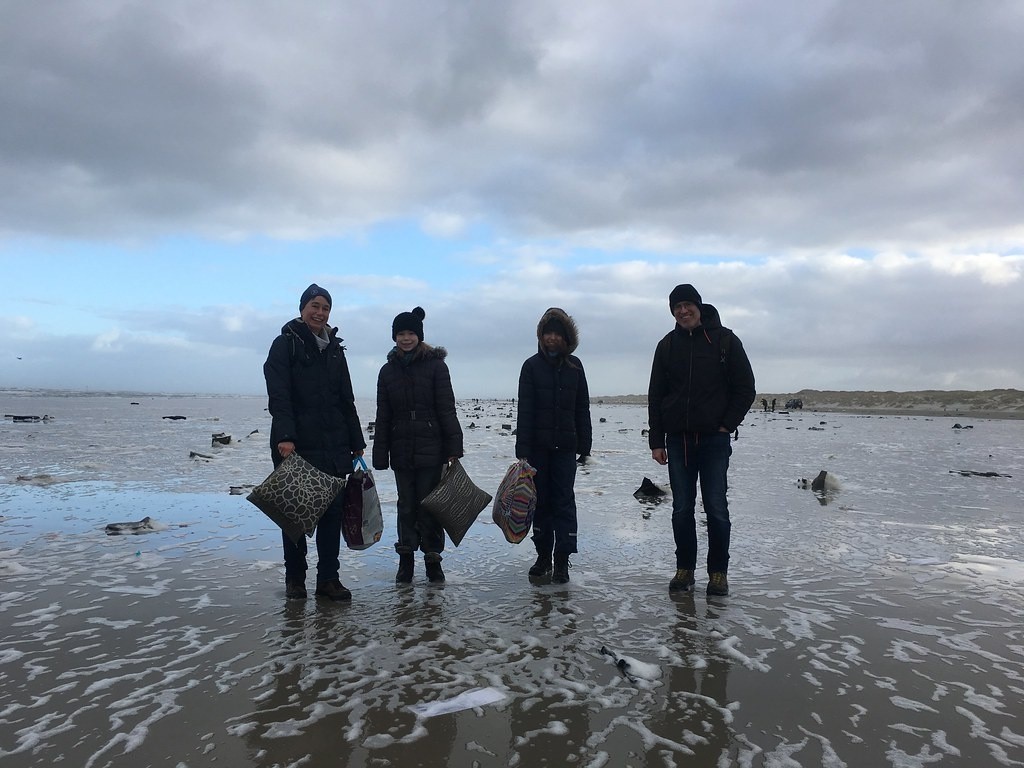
[669,284,702,316]
[391,307,425,341]
[300,283,331,313]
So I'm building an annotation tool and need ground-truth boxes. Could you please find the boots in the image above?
[394,543,414,583]
[424,551,445,582]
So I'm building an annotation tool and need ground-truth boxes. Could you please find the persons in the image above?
[647,284,756,594]
[772,399,776,411]
[515,307,592,584]
[372,306,465,582]
[263,283,366,599]
[761,399,767,411]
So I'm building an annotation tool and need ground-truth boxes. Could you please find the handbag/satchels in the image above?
[342,455,383,551]
[492,459,538,545]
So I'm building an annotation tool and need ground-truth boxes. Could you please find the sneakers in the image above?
[552,552,572,583]
[530,552,552,576]
[707,571,729,595]
[669,568,695,590]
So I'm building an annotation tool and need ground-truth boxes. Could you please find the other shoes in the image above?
[285,576,307,600]
[316,579,352,600]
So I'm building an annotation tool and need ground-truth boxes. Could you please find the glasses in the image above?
[672,301,694,312]
